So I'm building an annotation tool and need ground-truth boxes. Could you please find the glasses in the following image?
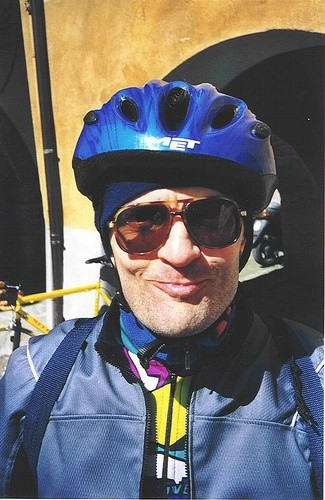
[103,196,250,257]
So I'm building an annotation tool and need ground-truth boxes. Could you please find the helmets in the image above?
[70,76,280,219]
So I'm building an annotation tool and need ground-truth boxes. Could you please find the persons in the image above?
[0,79,324,500]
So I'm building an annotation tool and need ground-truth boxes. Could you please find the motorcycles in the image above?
[251,202,282,265]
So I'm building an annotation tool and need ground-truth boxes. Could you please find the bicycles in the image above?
[0,252,114,379]
